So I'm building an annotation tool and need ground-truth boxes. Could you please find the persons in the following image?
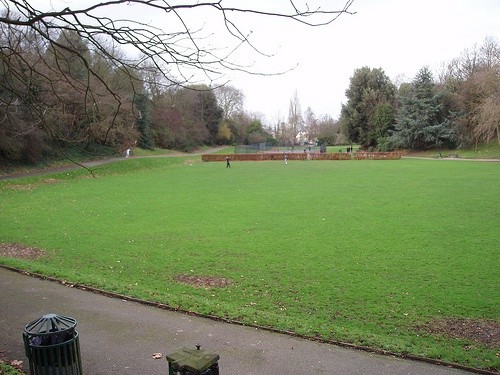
[226,157,231,167]
[284,154,288,164]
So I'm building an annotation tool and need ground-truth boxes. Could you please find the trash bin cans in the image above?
[22,313,85,375]
